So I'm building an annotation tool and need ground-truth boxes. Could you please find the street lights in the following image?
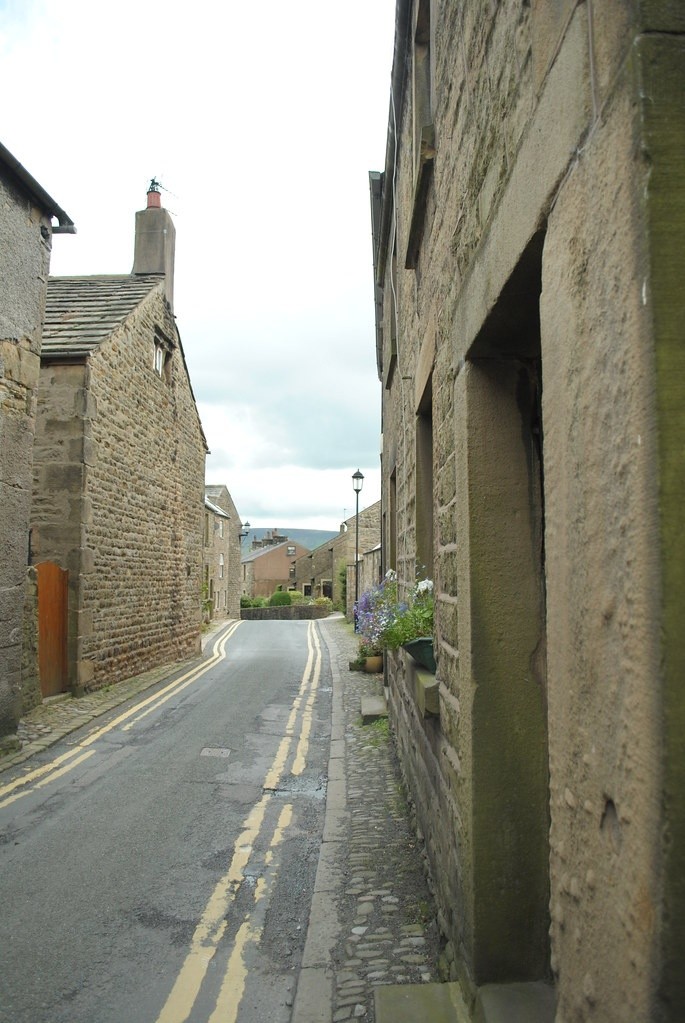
[351,469,364,634]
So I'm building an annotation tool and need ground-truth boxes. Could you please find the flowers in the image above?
[352,576,439,656]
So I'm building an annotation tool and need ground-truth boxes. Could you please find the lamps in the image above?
[238,521,252,544]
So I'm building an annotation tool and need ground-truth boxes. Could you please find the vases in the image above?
[400,636,436,673]
[364,656,383,674]
